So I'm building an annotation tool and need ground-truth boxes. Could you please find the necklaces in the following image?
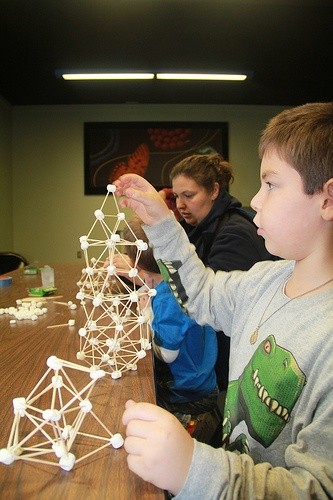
[250,272,333,345]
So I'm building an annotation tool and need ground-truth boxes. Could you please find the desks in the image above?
[0,261,166,500]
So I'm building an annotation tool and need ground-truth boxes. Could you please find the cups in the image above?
[41,268,54,290]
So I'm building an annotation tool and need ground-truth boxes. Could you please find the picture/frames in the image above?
[81,120,230,196]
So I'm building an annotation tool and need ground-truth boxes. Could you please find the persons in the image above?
[102,155,286,446]
[111,103,333,500]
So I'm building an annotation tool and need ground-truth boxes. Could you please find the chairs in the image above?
[0,252,27,275]
